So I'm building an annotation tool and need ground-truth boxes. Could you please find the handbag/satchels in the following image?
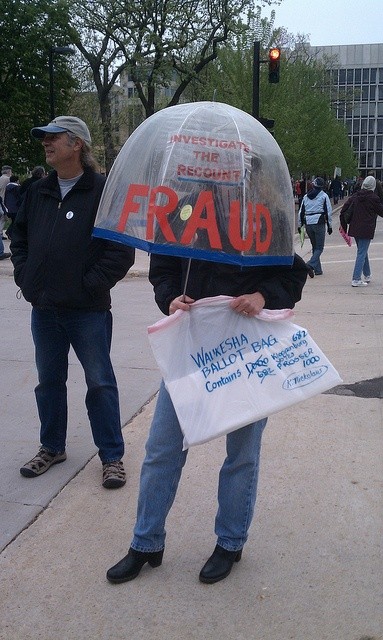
[342,201,354,224]
[338,224,353,247]
[0,201,8,215]
[300,224,305,248]
[147,295,344,451]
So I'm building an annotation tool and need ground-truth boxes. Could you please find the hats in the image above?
[207,123,264,165]
[2,165,12,170]
[312,177,325,187]
[31,116,92,146]
[361,176,376,192]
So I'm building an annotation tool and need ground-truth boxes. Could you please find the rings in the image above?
[242,310,248,315]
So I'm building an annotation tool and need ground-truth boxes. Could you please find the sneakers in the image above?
[102,460,127,488]
[352,279,368,287]
[305,264,314,278]
[315,271,322,275]
[364,274,372,282]
[2,236,7,239]
[6,231,13,240]
[0,253,11,260]
[20,449,66,477]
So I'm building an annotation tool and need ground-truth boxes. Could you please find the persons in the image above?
[105,122,307,586]
[4,175,21,239]
[7,116,134,488]
[375,178,383,197]
[0,165,11,240]
[0,195,10,261]
[340,176,383,287]
[297,177,332,278]
[21,166,44,196]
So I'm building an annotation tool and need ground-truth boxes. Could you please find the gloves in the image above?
[298,228,301,233]
[327,228,332,235]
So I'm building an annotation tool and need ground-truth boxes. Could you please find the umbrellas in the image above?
[91,101,296,306]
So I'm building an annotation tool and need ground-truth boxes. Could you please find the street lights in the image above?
[48,46,75,122]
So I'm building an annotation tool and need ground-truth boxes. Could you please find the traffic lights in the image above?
[268,48,279,83]
[262,117,274,137]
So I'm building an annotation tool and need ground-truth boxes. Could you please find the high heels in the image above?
[106,547,164,584]
[200,543,243,585]
